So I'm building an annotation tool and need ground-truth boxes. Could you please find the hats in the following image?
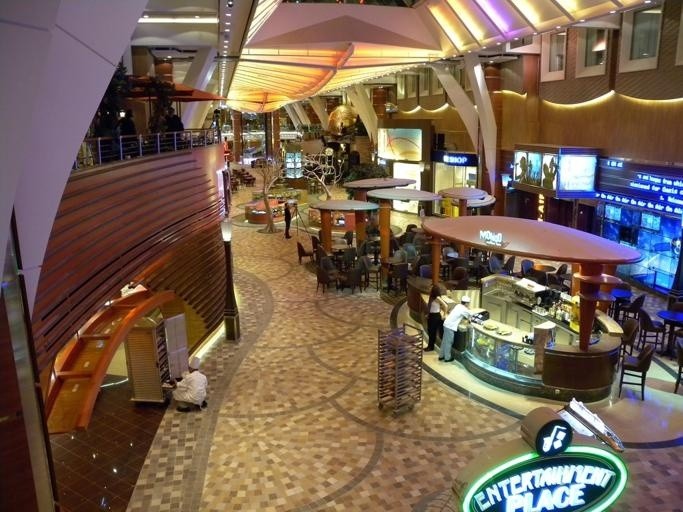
[189,357,200,370]
[462,296,470,302]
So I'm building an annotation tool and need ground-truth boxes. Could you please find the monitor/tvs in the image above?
[557,154,599,192]
[513,150,558,191]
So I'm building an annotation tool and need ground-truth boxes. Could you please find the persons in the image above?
[318,216,506,362]
[284,202,292,239]
[116,109,137,147]
[165,107,184,132]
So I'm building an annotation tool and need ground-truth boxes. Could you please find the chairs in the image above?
[442,246,569,292]
[296,224,436,298]
[604,281,683,402]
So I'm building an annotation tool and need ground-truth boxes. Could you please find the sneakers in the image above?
[423,346,434,351]
[196,401,207,408]
[439,356,454,362]
[176,406,190,412]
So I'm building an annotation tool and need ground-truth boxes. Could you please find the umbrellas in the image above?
[117,75,229,117]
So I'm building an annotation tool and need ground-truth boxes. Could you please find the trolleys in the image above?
[375,323,424,411]
